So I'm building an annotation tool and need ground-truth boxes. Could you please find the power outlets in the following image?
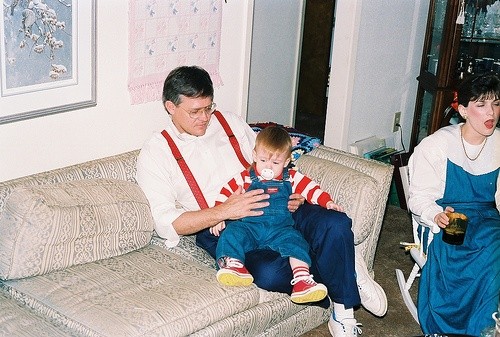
[393,111,401,133]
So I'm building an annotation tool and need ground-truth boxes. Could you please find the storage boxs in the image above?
[388,151,411,211]
[363,145,389,159]
[375,148,406,208]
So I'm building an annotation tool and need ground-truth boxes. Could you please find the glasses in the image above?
[177,99,218,119]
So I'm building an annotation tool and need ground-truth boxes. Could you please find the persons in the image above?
[208,124,340,305]
[130,65,388,337]
[405,71,500,337]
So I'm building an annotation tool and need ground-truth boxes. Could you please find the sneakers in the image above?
[354,254,388,317]
[327,308,363,337]
[217,259,254,288]
[290,275,327,304]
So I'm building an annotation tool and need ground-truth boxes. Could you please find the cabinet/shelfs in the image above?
[409,0,500,155]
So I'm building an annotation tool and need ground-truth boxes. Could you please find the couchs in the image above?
[0,144,395,337]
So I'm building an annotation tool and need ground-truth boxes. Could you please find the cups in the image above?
[442,211,468,245]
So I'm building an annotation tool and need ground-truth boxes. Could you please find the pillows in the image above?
[0,177,154,281]
[295,155,379,245]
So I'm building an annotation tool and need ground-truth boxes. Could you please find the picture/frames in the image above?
[0,0,97,125]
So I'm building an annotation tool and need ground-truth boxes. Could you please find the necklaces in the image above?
[460,124,488,162]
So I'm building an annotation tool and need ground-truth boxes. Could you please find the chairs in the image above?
[395,165,427,327]
